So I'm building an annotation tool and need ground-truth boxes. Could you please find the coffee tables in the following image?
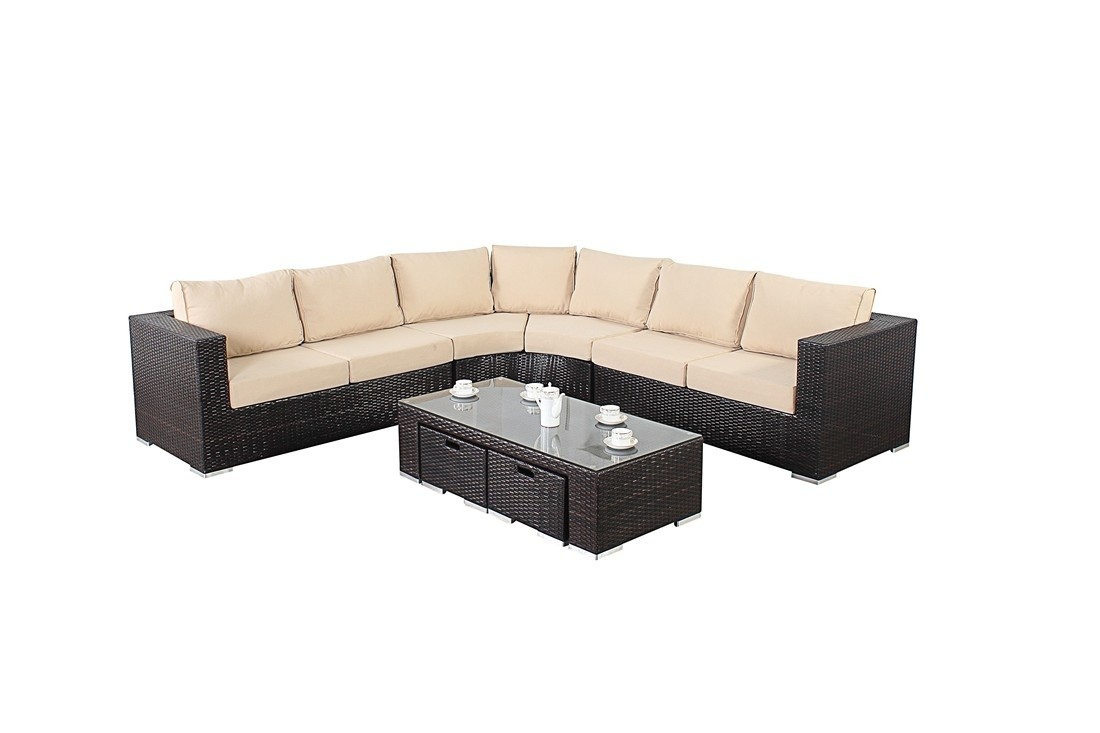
[399,374,707,558]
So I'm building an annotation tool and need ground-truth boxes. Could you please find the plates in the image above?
[595,413,628,425]
[449,387,479,398]
[603,436,638,449]
[519,391,536,401]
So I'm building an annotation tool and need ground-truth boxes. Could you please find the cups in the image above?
[453,379,472,395]
[525,383,544,399]
[612,428,633,446]
[599,405,620,421]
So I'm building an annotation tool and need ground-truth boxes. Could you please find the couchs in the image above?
[130,242,920,487]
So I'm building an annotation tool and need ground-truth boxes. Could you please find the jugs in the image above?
[536,382,565,428]
